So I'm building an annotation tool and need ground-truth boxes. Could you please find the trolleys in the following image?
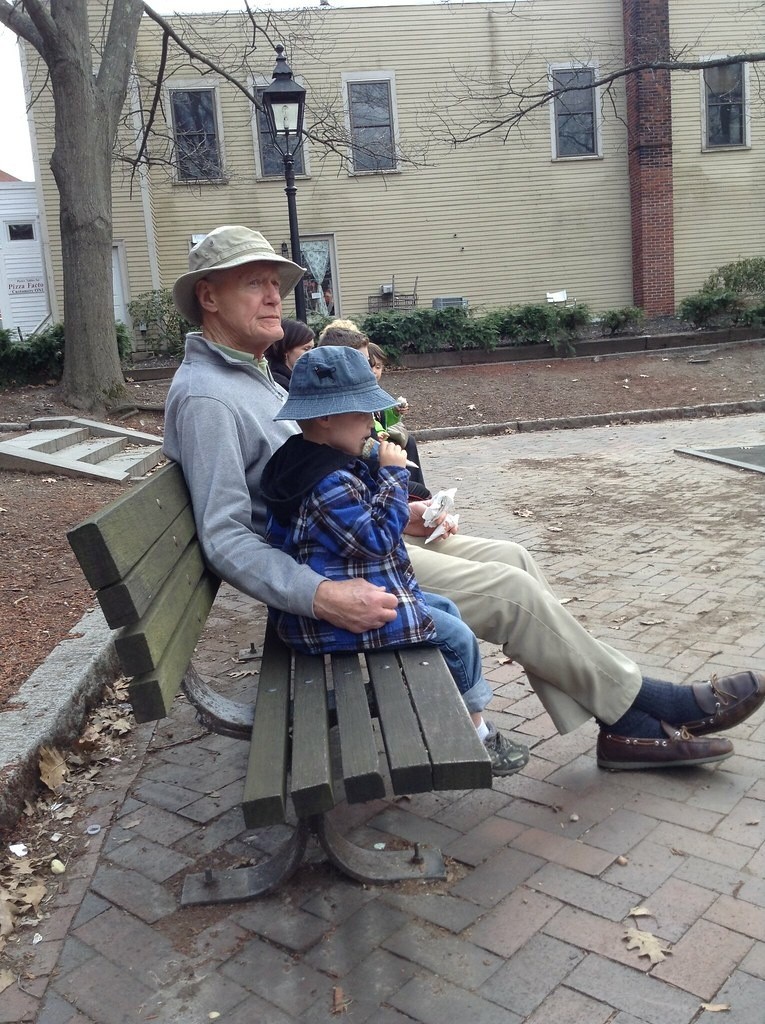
[368,275,419,314]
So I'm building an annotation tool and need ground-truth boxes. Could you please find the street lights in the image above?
[263,43,307,325]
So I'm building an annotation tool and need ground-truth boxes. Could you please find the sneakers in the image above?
[481,720,530,778]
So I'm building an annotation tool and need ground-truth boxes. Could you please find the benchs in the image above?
[66,461,494,907]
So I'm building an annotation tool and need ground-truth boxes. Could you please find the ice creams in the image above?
[361,437,419,468]
[396,395,407,408]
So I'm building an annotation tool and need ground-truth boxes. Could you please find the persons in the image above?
[318,318,433,502]
[164,226,765,769]
[262,318,316,392]
[261,344,530,778]
[365,341,426,485]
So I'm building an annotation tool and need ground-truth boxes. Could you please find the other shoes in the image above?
[672,668,765,736]
[597,720,735,768]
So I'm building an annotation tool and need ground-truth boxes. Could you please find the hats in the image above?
[273,344,401,422]
[172,225,307,327]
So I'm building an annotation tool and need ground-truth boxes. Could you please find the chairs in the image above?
[546,290,576,310]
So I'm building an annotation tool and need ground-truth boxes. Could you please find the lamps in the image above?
[281,242,289,259]
